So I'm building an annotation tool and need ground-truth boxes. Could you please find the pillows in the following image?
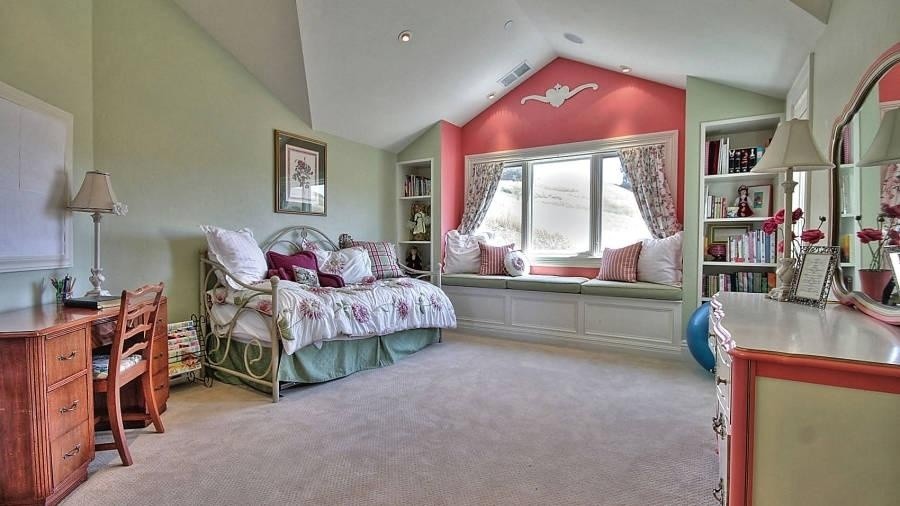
[441,231,530,277]
[598,232,683,290]
[200,225,400,288]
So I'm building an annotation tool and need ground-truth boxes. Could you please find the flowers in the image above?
[762,208,821,265]
[854,204,900,271]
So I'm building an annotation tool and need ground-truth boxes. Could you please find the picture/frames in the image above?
[786,246,839,309]
[275,129,328,215]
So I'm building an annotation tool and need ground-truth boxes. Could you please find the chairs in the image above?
[92,282,165,466]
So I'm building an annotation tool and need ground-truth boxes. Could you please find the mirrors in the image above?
[828,42,899,326]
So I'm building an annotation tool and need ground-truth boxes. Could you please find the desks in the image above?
[0,296,169,506]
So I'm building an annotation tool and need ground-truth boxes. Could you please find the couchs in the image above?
[198,227,457,404]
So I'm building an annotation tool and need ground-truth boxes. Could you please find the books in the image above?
[702,135,777,308]
[166,320,202,377]
[404,175,431,196]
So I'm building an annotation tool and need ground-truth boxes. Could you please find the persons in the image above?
[405,246,423,280]
[409,201,431,240]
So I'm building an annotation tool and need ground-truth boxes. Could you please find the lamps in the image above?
[855,109,899,167]
[750,117,836,300]
[68,170,127,296]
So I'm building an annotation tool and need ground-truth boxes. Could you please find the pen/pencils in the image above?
[51,273,76,304]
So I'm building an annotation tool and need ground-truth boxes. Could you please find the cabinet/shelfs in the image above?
[698,111,783,310]
[835,112,861,293]
[706,289,899,504]
[396,159,435,284]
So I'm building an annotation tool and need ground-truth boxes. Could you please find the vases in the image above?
[860,269,891,303]
